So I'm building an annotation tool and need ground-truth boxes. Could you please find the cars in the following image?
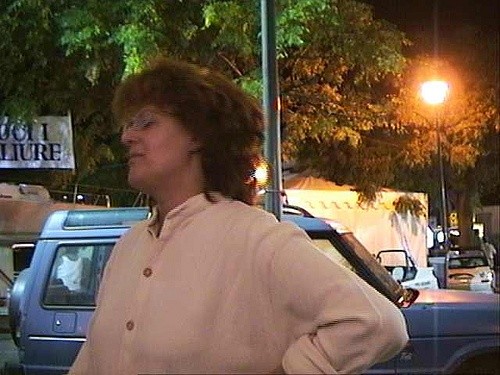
[373,248,438,291]
[440,250,494,291]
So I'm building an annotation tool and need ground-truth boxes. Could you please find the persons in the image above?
[49,245,93,294]
[481,235,497,271]
[66,60,408,375]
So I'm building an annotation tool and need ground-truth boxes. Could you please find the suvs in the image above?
[7,204,500,375]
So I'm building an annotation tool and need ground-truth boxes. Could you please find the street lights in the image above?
[421,79,455,253]
